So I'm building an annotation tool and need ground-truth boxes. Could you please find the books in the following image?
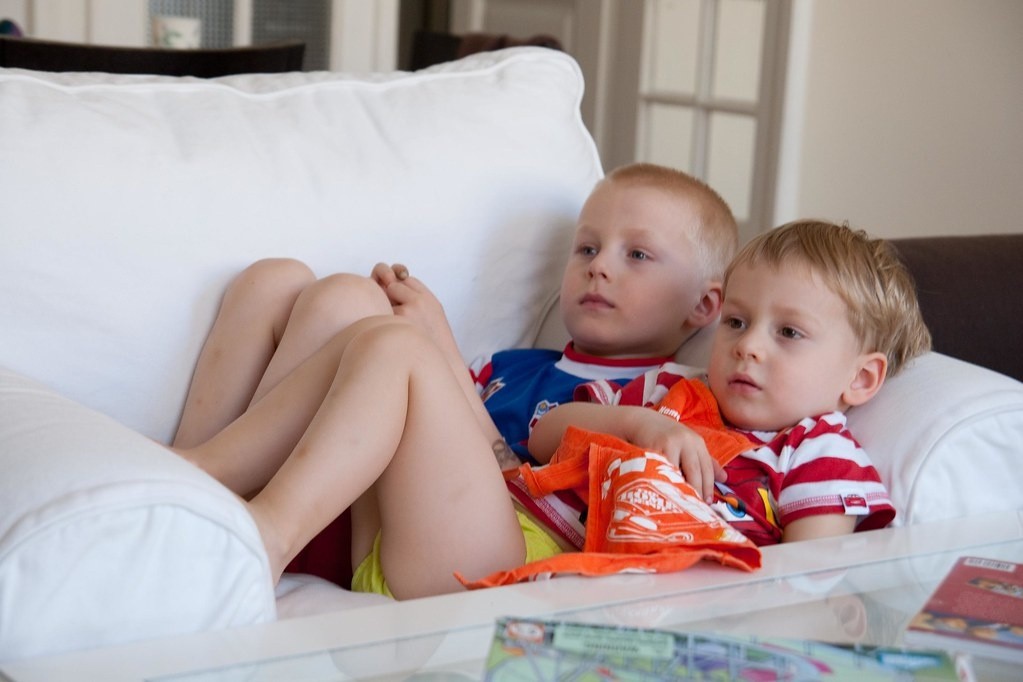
[906,555,1023,664]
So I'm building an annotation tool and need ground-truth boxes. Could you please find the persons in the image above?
[171,164,737,590]
[153,218,933,604]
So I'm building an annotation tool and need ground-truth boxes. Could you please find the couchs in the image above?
[0,45,1023,682]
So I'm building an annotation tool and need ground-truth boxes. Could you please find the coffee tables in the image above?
[149,535,1023,682]
